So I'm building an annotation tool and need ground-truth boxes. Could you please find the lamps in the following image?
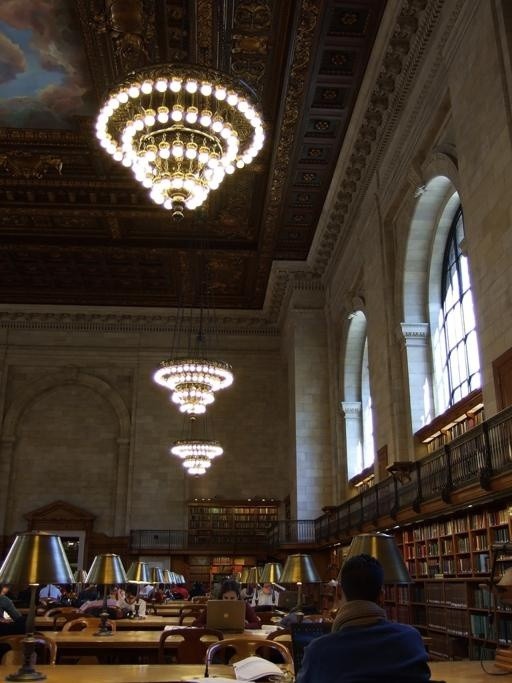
[152,190,236,479]
[94,0,266,225]
[0,530,186,682]
[232,532,410,615]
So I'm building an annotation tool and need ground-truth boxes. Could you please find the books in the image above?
[189,555,258,582]
[187,657,284,683]
[331,409,512,666]
[191,507,278,544]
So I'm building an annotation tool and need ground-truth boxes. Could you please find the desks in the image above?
[0,594,512,683]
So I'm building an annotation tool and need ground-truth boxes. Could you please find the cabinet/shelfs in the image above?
[321,389,512,661]
[188,499,281,542]
[189,554,284,599]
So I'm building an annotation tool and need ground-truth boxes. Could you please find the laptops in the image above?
[277,591,297,608]
[205,599,247,633]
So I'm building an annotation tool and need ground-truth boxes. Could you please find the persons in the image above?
[251,582,279,607]
[193,581,262,629]
[0,581,191,633]
[294,553,431,683]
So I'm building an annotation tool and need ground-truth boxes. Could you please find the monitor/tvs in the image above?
[290,622,333,681]
